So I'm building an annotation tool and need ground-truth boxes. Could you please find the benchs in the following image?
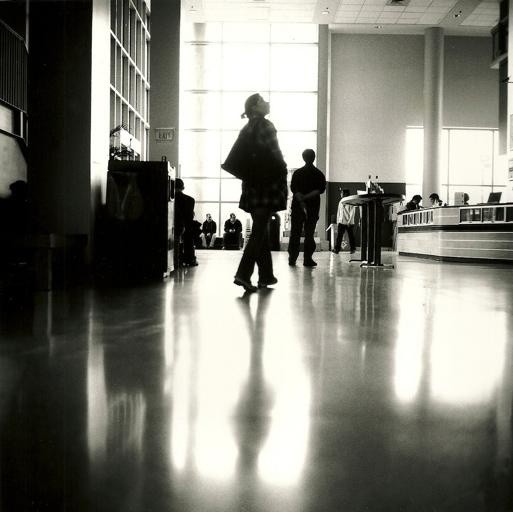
[195,236,244,250]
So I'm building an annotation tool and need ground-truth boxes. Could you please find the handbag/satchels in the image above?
[221,134,268,182]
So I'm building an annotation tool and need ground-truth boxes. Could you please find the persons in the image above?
[288,149,327,266]
[233,94,288,292]
[429,193,443,206]
[174,178,242,266]
[406,194,422,211]
[332,190,356,254]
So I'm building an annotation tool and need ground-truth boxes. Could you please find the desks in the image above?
[341,193,406,269]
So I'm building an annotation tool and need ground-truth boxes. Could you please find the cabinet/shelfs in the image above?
[107,160,176,280]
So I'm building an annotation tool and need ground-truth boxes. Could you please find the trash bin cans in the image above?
[36,233,63,290]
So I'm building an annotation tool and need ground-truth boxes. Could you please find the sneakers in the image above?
[303,260,317,266]
[233,278,257,290]
[289,260,295,265]
[259,277,277,287]
